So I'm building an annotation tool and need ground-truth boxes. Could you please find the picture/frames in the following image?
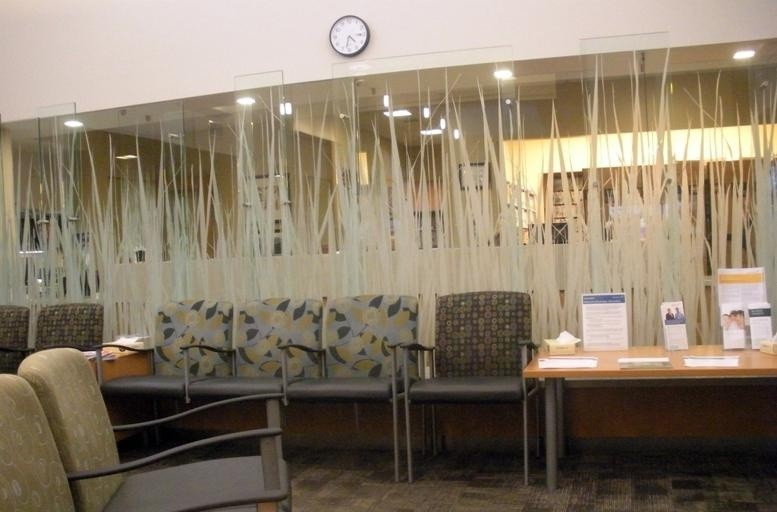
[240,171,292,236]
[456,162,493,191]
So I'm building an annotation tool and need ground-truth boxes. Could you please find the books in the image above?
[537,266,773,370]
[81,336,145,363]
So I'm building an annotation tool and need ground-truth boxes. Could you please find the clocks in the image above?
[328,14,370,59]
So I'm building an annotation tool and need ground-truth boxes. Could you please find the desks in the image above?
[522,343,775,491]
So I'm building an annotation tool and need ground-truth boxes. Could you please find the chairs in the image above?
[1,302,32,374]
[18,346,292,511]
[91,298,232,463]
[187,295,324,459]
[399,290,541,486]
[1,371,81,511]
[31,301,106,352]
[283,293,428,484]
